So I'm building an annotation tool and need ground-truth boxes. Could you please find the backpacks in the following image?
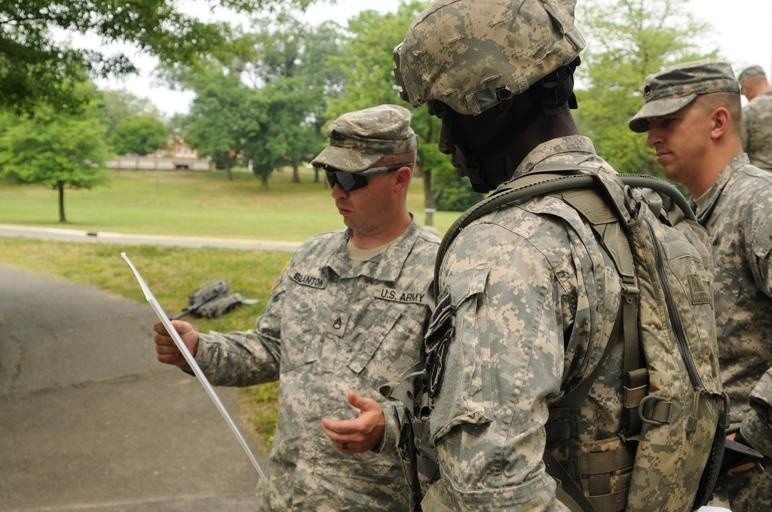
[485,162,732,512]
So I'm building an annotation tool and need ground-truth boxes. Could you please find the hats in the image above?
[738,65,765,95]
[311,104,420,173]
[629,61,740,133]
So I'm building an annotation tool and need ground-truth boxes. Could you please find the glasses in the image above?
[322,162,415,193]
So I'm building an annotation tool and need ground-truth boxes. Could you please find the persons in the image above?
[627,61,772,512]
[380,0,730,511]
[153,105,448,511]
[738,65,771,173]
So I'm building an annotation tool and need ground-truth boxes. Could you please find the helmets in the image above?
[392,0,586,118]
